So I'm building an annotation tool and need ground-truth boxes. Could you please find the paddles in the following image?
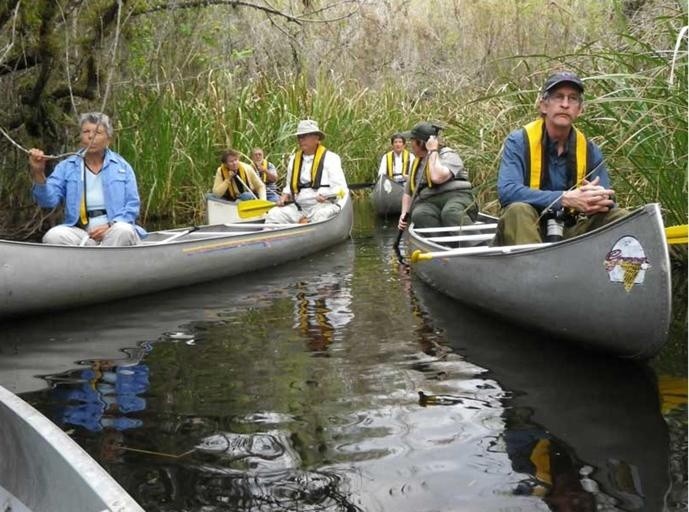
[238,190,344,219]
[409,223,688,264]
[347,179,408,190]
[394,126,444,248]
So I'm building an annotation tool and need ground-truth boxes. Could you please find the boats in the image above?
[408,201,673,363]
[369,173,405,219]
[0,191,355,319]
[1,386,147,512]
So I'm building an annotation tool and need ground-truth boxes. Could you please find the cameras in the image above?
[540,206,577,242]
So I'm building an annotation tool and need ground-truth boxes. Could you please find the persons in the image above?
[489,72,632,249]
[213,150,269,202]
[250,147,278,198]
[26,113,148,247]
[410,307,464,409]
[378,132,416,190]
[398,123,491,251]
[293,272,355,355]
[263,121,349,235]
[499,407,598,510]
[63,347,159,465]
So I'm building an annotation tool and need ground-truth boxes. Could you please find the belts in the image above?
[87,210,106,217]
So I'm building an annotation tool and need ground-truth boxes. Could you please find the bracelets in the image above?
[426,149,439,155]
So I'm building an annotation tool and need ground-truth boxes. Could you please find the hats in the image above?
[542,71,584,92]
[399,121,437,142]
[293,120,326,140]
